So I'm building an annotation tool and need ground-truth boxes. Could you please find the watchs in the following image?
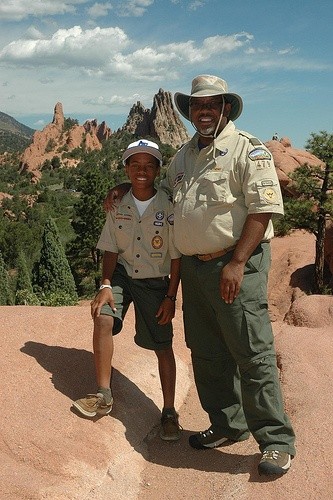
[99,284,112,290]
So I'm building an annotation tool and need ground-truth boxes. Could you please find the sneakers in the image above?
[257,450,291,474]
[73,393,113,417]
[160,412,183,440]
[189,428,228,449]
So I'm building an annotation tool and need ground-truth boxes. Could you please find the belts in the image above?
[193,246,237,261]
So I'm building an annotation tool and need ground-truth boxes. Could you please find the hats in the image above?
[122,139,163,167]
[174,74,243,121]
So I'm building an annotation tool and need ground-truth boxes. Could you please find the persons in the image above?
[72,138,183,440]
[104,75,295,475]
[271,132,280,141]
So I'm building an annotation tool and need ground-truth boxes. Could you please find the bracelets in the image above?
[165,293,176,302]
